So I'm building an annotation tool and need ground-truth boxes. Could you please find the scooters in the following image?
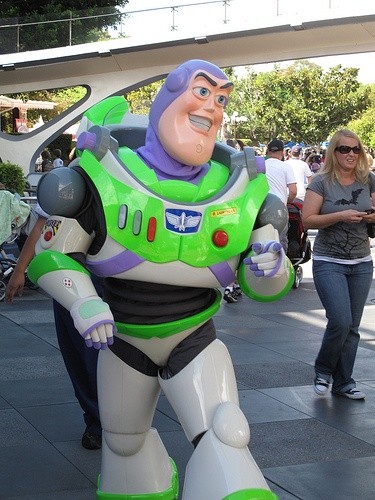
[0,222,40,292]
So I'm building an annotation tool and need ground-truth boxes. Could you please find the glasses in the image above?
[335,145,360,155]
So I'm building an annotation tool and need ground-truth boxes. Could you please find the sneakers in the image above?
[314,376,330,396]
[341,386,366,399]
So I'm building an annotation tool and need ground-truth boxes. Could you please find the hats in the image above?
[267,139,284,152]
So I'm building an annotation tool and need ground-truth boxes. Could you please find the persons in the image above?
[32,59,297,500]
[0,130,375,302]
[300,133,375,401]
[5,197,102,449]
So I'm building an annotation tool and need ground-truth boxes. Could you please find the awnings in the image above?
[0,96,56,110]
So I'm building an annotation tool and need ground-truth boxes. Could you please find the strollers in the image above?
[286,197,312,289]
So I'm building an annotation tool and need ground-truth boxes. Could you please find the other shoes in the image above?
[222,288,238,303]
[233,286,243,296]
[82,430,102,449]
